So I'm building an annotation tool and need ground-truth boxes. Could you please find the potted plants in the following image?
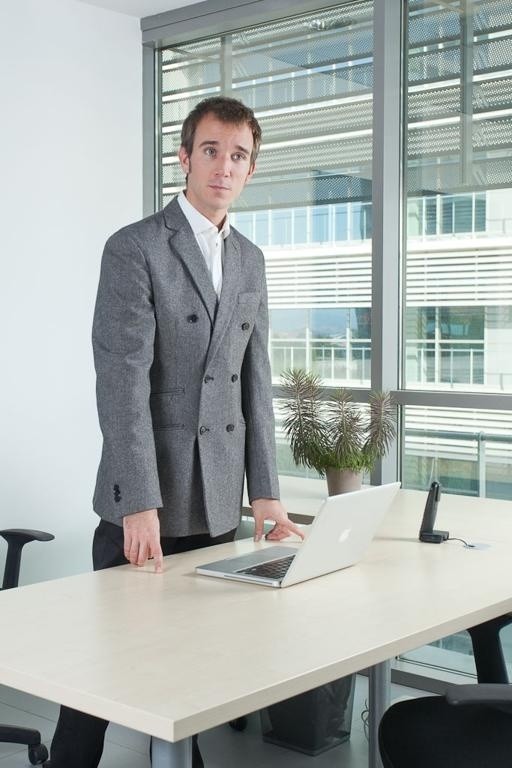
[273,365,400,498]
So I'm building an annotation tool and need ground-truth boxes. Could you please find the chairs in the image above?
[378,616,511,767]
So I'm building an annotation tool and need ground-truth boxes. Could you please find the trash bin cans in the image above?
[259,672,356,757]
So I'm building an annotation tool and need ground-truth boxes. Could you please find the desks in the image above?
[0,489,511,767]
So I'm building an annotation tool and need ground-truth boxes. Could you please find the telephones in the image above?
[418,481,449,542]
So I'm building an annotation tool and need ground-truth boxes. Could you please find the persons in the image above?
[44,98,306,767]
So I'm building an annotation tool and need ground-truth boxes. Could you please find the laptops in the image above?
[195,481,400,589]
